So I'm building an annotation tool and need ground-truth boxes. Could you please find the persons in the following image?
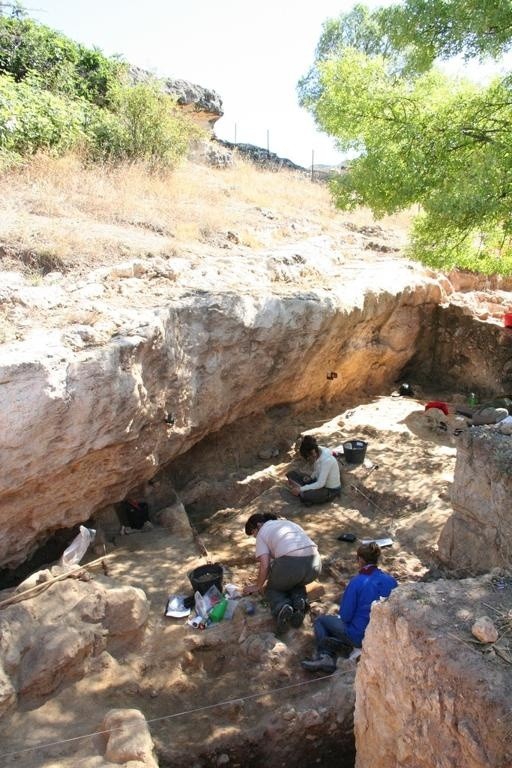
[285,434,344,506]
[301,539,401,674]
[243,512,324,631]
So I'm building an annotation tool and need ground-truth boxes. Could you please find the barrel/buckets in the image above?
[124,502,150,529]
[342,440,368,463]
[187,564,224,597]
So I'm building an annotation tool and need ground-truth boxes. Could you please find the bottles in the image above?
[210,595,229,621]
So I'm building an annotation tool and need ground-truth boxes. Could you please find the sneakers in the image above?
[300,656,336,673]
[277,604,294,633]
[348,647,361,663]
[290,598,306,628]
